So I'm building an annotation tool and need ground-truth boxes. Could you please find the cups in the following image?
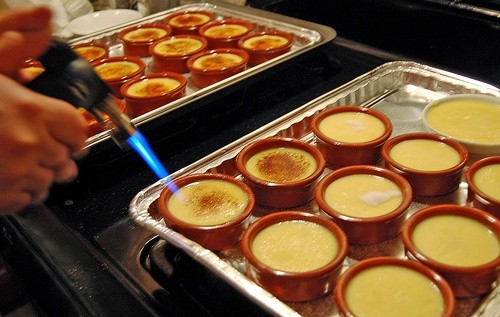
[156,173,255,252]
[380,132,469,198]
[118,24,174,57]
[313,165,414,246]
[237,30,294,67]
[199,18,255,49]
[235,137,326,209]
[333,255,455,317]
[17,62,44,81]
[120,72,187,117]
[186,47,250,89]
[239,211,349,304]
[400,204,499,298]
[89,56,147,100]
[149,34,208,73]
[163,10,217,36]
[311,104,394,166]
[465,155,500,217]
[78,97,127,136]
[70,44,109,65]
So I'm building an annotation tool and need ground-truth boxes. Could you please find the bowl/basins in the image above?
[420,94,500,155]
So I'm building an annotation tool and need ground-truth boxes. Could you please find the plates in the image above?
[66,8,143,37]
